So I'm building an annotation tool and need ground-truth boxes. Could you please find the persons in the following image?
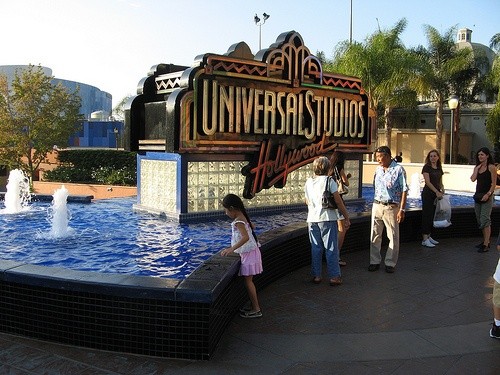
[304,157,349,286]
[366,146,408,273]
[221,194,263,318]
[329,151,349,266]
[489,234,500,339]
[394,152,403,163]
[470,148,497,252]
[421,150,445,247]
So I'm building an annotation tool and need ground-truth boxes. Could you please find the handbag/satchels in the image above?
[334,166,348,194]
[433,194,452,228]
[322,177,345,209]
[473,192,492,203]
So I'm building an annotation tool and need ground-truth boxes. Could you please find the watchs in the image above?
[400,208,406,213]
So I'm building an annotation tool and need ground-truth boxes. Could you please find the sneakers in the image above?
[368,264,379,271]
[240,310,262,318]
[428,234,439,244]
[386,266,396,273]
[479,245,488,252]
[240,306,252,312]
[476,242,490,248]
[422,239,435,247]
[489,322,500,339]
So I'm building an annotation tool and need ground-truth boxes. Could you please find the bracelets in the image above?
[487,192,490,197]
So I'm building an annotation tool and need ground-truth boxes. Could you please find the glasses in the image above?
[378,149,385,152]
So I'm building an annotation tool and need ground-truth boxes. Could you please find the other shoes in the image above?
[496,245,500,250]
[330,277,343,286]
[339,258,346,266]
[312,276,321,283]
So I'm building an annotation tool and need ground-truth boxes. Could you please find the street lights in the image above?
[447,95,459,164]
[114,128,119,150]
[254,13,270,51]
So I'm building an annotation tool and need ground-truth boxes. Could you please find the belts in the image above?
[374,199,396,205]
